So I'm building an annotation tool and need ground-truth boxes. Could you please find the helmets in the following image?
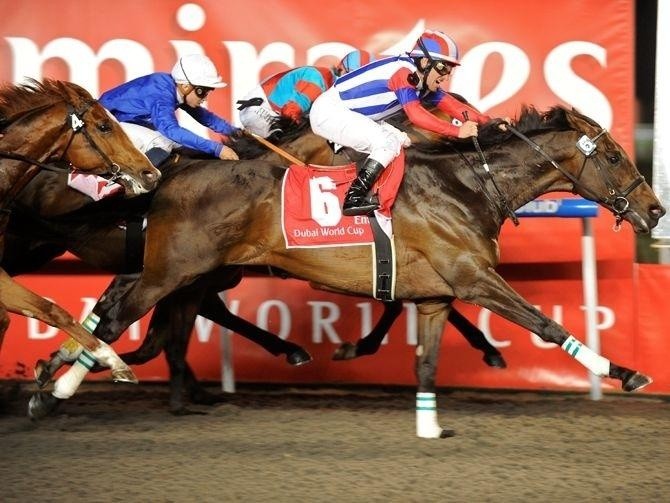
[410,29,461,65]
[339,50,377,70]
[171,54,228,88]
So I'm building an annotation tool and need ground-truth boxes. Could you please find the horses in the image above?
[27,103,666,439]
[50,90,506,404]
[0,74,226,405]
[0,76,162,384]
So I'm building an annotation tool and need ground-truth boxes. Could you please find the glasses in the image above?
[433,61,451,76]
[195,87,214,97]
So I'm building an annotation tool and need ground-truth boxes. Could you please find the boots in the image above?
[343,158,385,214]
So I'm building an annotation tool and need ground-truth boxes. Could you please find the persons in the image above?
[240,48,377,150]
[96,53,245,169]
[309,28,518,216]
